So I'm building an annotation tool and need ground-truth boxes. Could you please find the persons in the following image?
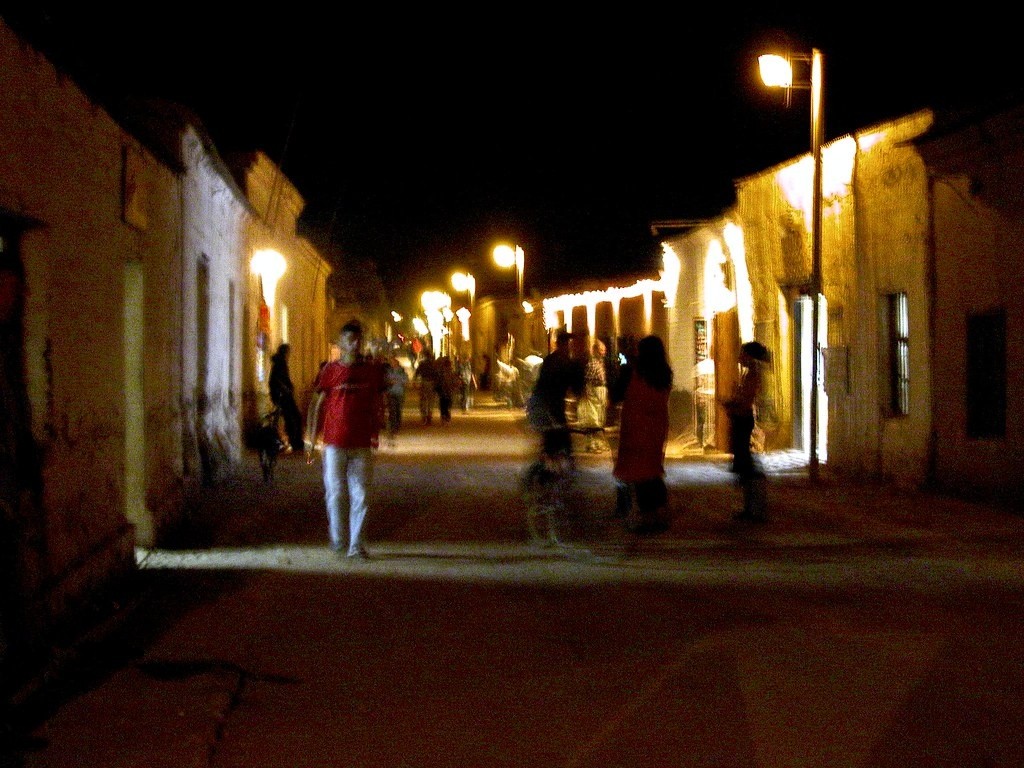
[723,342,773,520]
[610,335,674,514]
[269,344,303,450]
[388,336,479,432]
[306,321,388,558]
[518,331,587,491]
[576,340,610,454]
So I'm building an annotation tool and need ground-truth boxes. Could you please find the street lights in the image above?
[493,244,525,312]
[757,48,825,487]
